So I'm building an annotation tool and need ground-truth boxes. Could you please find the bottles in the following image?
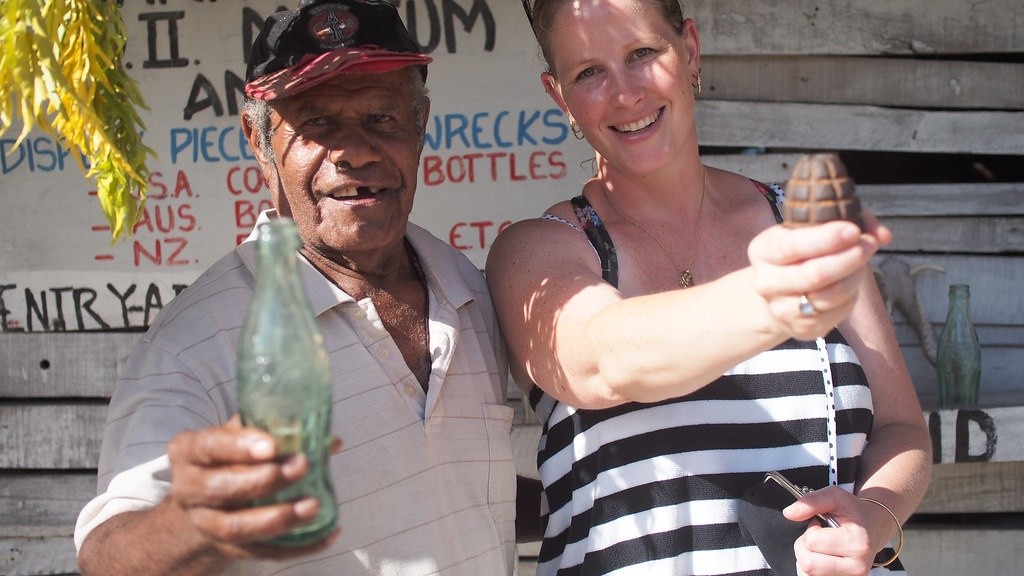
[240,221,337,548]
[937,285,982,410]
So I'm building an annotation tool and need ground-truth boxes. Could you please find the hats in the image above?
[245,1,432,102]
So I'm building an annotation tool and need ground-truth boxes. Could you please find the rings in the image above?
[798,295,820,318]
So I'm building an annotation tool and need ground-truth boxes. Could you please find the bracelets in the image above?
[856,496,904,570]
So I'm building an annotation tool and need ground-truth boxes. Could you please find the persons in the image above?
[484,0,934,576]
[73,0,518,576]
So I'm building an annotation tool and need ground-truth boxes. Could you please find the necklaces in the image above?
[598,164,706,290]
